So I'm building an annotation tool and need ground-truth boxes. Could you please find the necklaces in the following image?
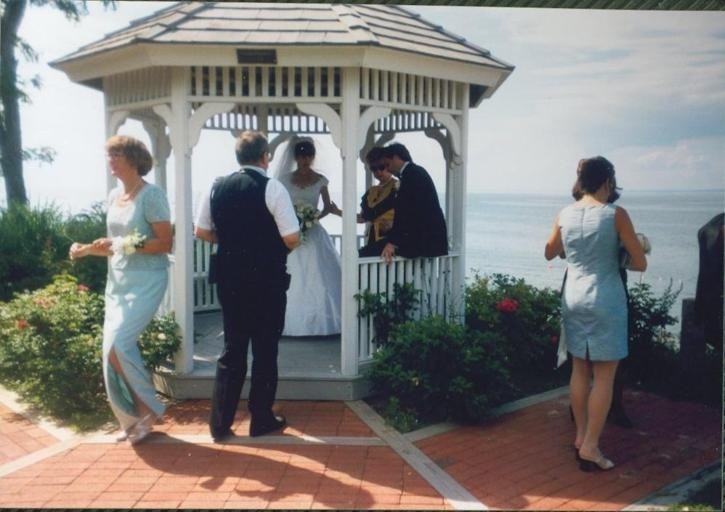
[115,180,145,201]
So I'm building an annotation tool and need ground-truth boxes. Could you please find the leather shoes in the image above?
[209,424,235,440]
[248,415,287,437]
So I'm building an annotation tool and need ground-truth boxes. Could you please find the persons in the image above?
[562,162,640,427]
[359,142,450,266]
[195,130,301,444]
[268,134,343,338]
[543,155,651,472]
[328,147,400,247]
[69,132,173,448]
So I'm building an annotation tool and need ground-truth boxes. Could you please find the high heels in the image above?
[129,411,155,444]
[573,444,583,456]
[579,452,615,471]
[116,421,137,442]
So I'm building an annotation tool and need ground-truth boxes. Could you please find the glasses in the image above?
[368,164,385,171]
[105,151,125,160]
